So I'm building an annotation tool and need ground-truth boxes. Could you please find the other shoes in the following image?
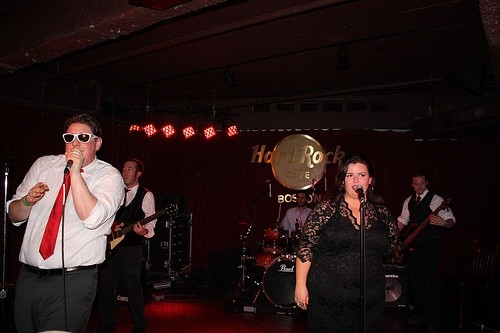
[132,326,144,333]
[95,318,114,333]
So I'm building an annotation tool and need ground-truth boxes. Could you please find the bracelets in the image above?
[22,197,29,207]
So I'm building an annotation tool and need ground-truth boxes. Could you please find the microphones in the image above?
[64,160,73,174]
[357,185,365,205]
[269,182,272,197]
[312,179,314,196]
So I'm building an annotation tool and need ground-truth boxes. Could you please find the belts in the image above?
[17,261,99,280]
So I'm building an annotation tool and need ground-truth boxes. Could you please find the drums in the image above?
[263,254,299,309]
[252,242,287,270]
[291,228,302,247]
[261,226,288,247]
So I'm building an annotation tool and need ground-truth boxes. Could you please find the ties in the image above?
[122,188,131,206]
[416,196,421,205]
[39,168,84,261]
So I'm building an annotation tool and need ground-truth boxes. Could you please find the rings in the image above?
[299,302,302,305]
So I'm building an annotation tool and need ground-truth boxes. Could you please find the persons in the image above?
[294,154,406,333]
[279,190,313,238]
[395,169,457,275]
[4,113,126,333]
[95,159,157,333]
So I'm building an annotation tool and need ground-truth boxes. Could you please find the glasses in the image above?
[63,133,98,144]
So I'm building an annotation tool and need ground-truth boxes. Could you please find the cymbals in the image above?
[237,221,265,231]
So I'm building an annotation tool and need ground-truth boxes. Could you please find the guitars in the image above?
[107,201,179,258]
[394,197,452,267]
